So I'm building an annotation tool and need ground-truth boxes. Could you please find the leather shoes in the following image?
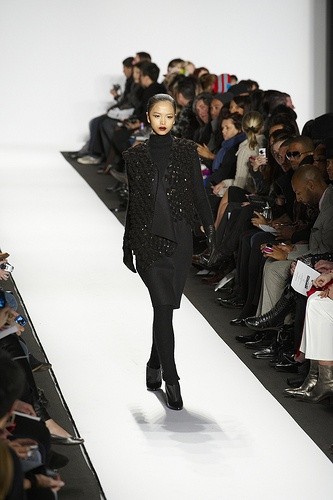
[77,154,236,302]
[269,353,296,364]
[230,318,241,326]
[275,358,308,373]
[50,436,84,446]
[235,331,268,343]
[245,338,272,351]
[239,318,248,329]
[219,298,242,308]
[287,374,308,389]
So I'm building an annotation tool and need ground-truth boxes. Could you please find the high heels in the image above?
[165,380,183,411]
[146,364,162,391]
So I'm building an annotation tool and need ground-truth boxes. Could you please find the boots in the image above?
[284,359,321,398]
[252,329,294,358]
[294,364,333,403]
[244,289,299,331]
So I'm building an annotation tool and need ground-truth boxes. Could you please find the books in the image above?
[259,224,280,236]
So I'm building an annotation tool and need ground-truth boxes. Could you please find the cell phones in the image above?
[259,148,266,159]
[14,314,27,326]
[265,242,273,250]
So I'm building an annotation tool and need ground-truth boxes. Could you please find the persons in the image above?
[122,94,216,409]
[0,250,84,500]
[229,113,333,403]
[70,53,164,212]
[161,58,294,308]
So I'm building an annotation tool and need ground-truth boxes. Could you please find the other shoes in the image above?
[38,389,49,405]
[28,353,51,372]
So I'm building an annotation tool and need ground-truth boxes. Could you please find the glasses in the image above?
[286,150,315,161]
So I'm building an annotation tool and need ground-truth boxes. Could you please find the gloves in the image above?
[207,235,218,267]
[123,249,136,273]
